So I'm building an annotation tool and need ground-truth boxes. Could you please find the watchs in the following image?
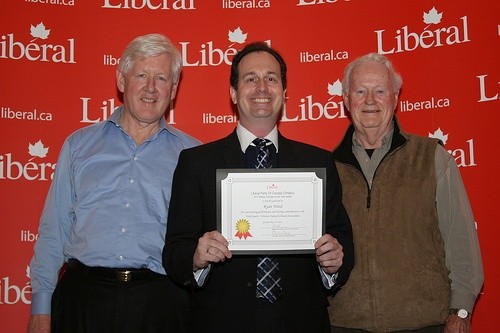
[449,308,471,320]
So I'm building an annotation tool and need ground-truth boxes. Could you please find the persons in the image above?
[161,41,354,333]
[28,33,204,333]
[327,53,483,333]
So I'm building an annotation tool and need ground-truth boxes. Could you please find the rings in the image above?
[207,245,212,253]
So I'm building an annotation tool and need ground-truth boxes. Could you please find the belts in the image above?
[73,260,156,283]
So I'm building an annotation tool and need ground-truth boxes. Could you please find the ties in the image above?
[252,137,284,305]
